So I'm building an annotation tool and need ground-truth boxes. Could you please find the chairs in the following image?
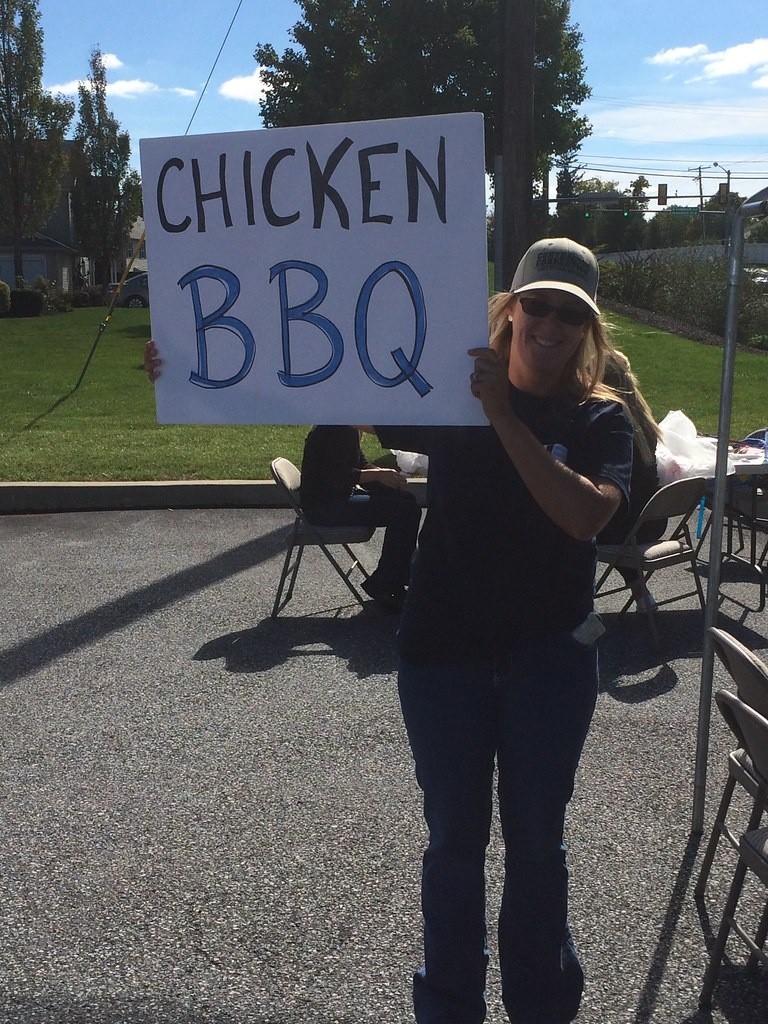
[268,457,370,618]
[693,429,768,565]
[693,626,768,935]
[698,688,768,1009]
[592,477,707,621]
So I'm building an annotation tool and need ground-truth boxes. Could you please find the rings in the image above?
[474,371,478,382]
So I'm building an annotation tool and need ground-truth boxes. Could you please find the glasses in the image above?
[516,296,590,327]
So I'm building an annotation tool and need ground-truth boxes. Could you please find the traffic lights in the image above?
[623,202,629,216]
[584,204,590,218]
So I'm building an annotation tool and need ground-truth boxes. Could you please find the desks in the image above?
[405,445,768,604]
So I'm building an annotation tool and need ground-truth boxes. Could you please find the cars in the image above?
[104,272,149,309]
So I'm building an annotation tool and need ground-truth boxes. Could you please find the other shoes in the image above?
[361,573,405,610]
[635,595,657,616]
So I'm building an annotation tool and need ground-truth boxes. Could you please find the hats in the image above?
[509,238,602,316]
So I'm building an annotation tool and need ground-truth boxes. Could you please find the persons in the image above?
[299,423,421,614]
[588,346,667,612]
[144,237,635,1024]
